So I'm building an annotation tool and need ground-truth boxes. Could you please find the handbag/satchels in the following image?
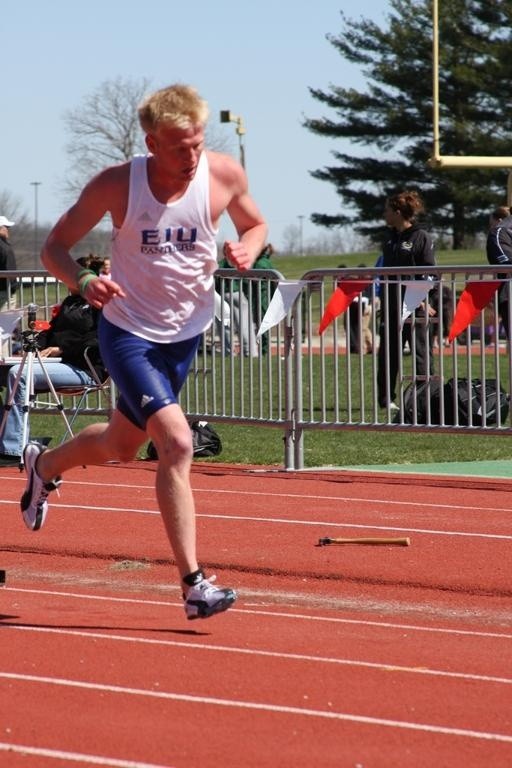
[147,421,222,460]
[394,375,510,426]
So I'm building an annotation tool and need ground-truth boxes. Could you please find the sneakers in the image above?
[382,402,400,414]
[182,574,238,620]
[0,453,21,467]
[21,441,64,531]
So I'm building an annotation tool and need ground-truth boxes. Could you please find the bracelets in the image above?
[72,268,98,285]
[75,273,97,297]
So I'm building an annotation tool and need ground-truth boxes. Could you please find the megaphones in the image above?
[220,110,241,126]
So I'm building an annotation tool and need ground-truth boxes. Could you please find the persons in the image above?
[1,253,107,467]
[487,207,510,227]
[216,241,254,357]
[429,281,453,349]
[100,257,112,278]
[376,190,436,411]
[248,243,275,358]
[333,253,383,354]
[0,217,21,358]
[20,83,268,621]
[487,206,512,339]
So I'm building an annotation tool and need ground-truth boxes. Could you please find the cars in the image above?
[451,288,508,346]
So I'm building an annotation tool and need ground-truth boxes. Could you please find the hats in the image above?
[0,215,15,227]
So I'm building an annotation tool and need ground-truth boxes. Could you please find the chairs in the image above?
[50,346,113,444]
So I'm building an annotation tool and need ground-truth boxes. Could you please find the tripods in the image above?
[0,342,87,472]
